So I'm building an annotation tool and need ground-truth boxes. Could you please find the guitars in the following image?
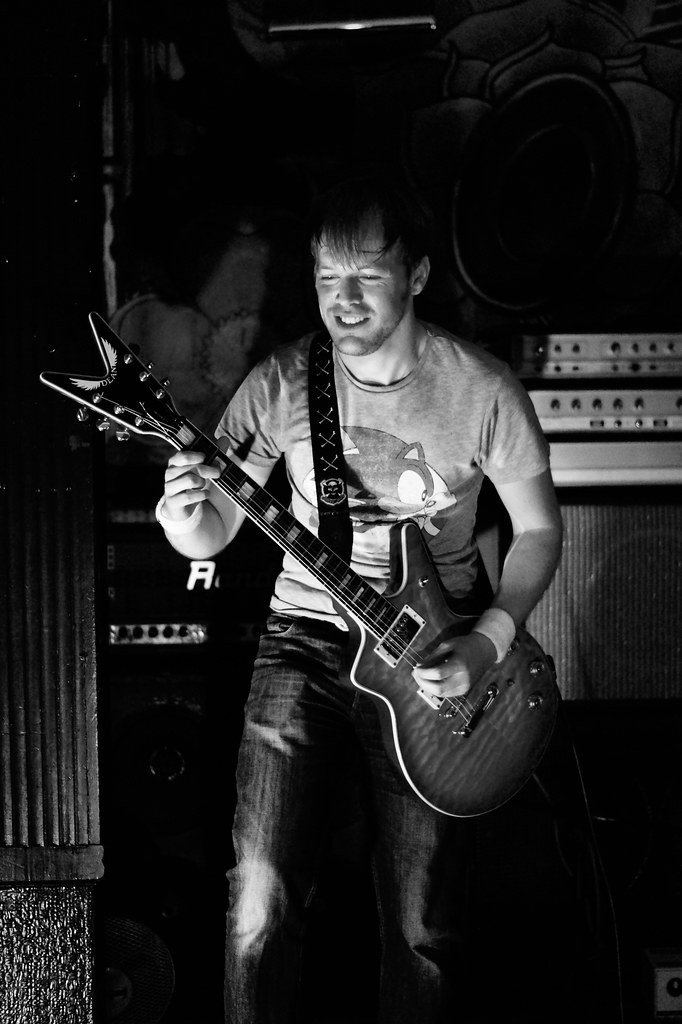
[38,306,563,822]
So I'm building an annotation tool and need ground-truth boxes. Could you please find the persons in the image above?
[155,493,203,535]
[153,180,565,1024]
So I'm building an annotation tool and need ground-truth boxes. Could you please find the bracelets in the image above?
[473,607,515,664]
